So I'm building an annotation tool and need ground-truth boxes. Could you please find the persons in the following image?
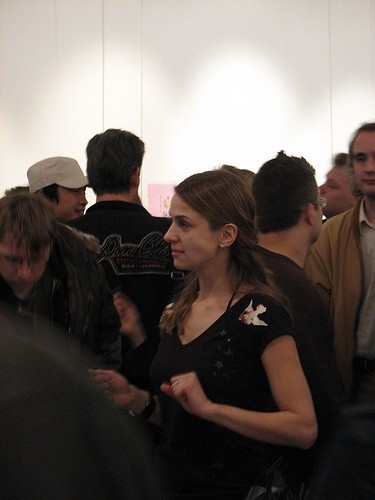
[0,122,375,500]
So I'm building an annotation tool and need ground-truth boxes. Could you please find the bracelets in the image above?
[129,390,156,422]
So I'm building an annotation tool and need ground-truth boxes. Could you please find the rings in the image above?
[176,379,179,385]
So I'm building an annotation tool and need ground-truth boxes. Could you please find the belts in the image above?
[352,356,375,374]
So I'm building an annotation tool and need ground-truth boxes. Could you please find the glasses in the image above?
[300,196,327,210]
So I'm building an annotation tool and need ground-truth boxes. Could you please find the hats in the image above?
[252,150,310,215]
[27,157,89,194]
